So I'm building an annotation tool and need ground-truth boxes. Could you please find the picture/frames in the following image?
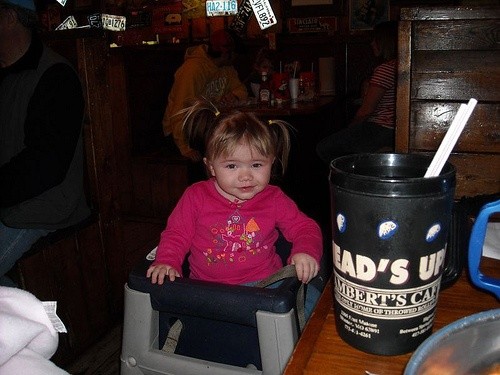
[348,0,390,32]
[284,0,343,16]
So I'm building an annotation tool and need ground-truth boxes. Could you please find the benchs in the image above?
[13,37,190,368]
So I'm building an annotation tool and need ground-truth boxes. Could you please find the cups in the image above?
[467,200,500,300]
[329,153,464,356]
[288,78,299,99]
[318,58,334,96]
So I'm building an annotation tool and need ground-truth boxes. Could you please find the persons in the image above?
[316,20,399,166]
[163,28,281,162]
[0,0,92,287]
[146,97,326,318]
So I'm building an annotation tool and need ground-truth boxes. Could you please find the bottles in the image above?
[299,76,304,94]
[259,72,272,103]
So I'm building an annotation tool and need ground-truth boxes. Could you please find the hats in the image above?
[210,30,240,51]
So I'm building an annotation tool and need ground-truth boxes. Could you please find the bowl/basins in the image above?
[404,308,500,375]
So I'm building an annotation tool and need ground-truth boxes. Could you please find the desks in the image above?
[282,256,500,375]
[246,97,336,115]
